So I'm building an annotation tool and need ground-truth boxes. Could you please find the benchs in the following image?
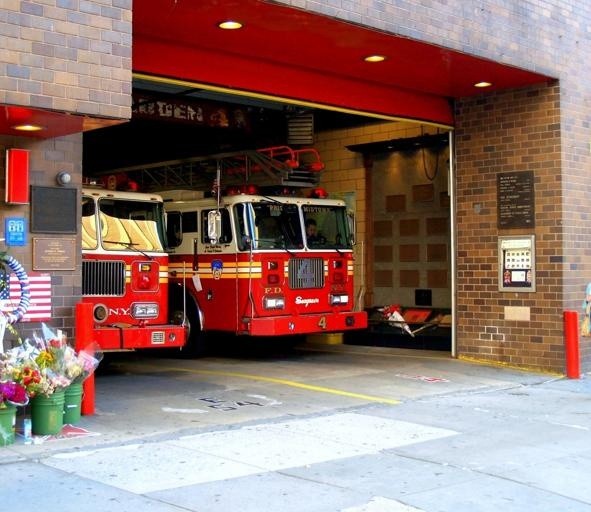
[362,303,452,350]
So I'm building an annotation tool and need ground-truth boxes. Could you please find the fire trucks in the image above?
[113,144,372,358]
[81,173,189,367]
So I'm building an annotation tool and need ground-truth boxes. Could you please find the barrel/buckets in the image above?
[65,383,86,426]
[0,405,17,447]
[32,390,65,435]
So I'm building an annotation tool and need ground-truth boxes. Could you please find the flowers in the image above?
[381,303,416,339]
[0,332,97,411]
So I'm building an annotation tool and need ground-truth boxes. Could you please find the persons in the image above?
[297,218,325,248]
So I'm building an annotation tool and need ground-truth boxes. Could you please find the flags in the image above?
[0,272,52,322]
[213,167,221,193]
